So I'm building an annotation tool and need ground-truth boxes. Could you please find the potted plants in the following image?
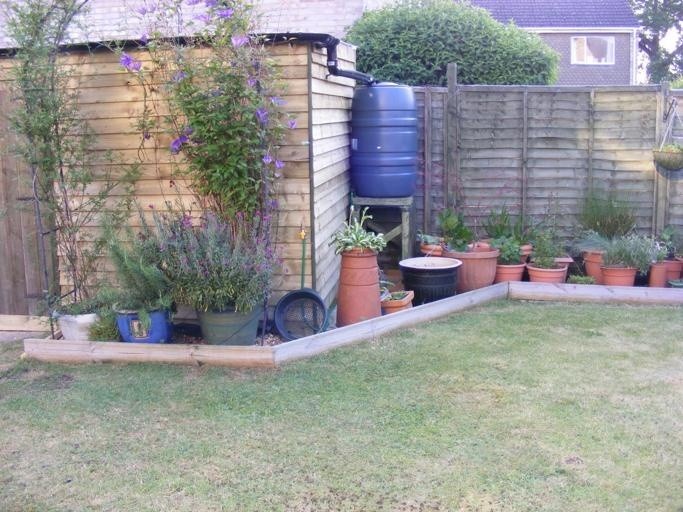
[378,284,415,315]
[393,238,463,310]
[99,220,174,343]
[0,0,146,343]
[525,191,575,286]
[328,202,389,326]
[574,182,665,289]
[645,246,682,289]
[417,207,530,290]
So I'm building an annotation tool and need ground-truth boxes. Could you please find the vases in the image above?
[195,307,258,346]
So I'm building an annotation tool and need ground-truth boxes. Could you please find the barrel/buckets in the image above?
[349,81,420,199]
[111,301,174,343]
[196,302,262,345]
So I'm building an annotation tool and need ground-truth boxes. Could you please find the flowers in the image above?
[137,171,287,315]
